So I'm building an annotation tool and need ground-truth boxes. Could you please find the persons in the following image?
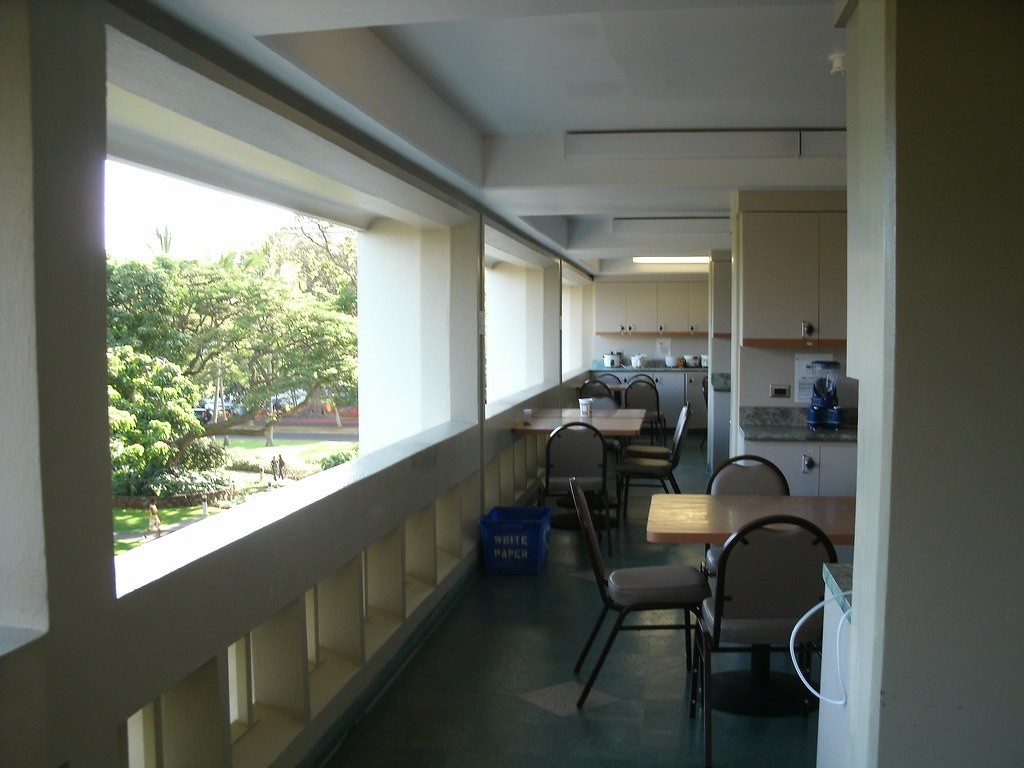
[271,454,284,481]
[143,499,161,539]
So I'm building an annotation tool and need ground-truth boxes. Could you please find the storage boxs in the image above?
[476,506,556,576]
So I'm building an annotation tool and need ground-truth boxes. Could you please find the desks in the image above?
[646,494,855,716]
[575,383,629,408]
[512,408,646,531]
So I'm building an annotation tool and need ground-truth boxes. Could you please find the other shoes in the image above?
[143,535,147,539]
[156,536,161,538]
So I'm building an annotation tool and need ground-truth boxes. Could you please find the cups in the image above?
[579,399,593,417]
[523,409,532,426]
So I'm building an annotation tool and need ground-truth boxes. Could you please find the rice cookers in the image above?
[603,352,621,368]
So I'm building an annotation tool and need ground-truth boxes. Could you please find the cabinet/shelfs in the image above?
[685,373,708,430]
[739,212,819,348]
[591,372,685,429]
[735,431,820,496]
[689,281,709,336]
[595,282,658,335]
[658,281,689,336]
[819,211,848,348]
[820,443,857,496]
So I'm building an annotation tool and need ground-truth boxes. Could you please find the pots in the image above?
[630,353,648,368]
[666,356,677,368]
[684,354,699,367]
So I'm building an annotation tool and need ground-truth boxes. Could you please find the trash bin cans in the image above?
[479,504,553,575]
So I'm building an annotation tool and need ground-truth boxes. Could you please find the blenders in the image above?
[807,361,840,429]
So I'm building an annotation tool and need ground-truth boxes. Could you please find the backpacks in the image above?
[281,460,285,466]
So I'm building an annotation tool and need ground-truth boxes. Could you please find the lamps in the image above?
[202,495,207,503]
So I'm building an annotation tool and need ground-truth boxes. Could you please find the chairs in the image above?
[537,374,838,768]
[700,377,708,448]
